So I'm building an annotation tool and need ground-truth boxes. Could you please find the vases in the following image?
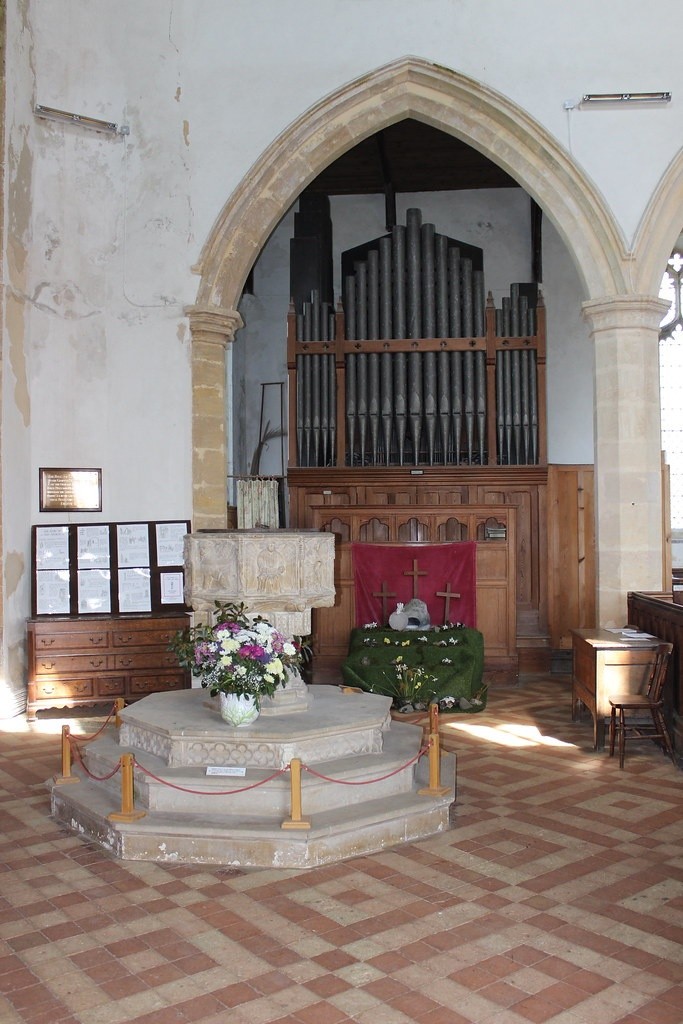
[394,696,411,709]
[219,690,261,727]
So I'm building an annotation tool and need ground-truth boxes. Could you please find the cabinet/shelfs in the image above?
[24,613,193,723]
[567,626,668,752]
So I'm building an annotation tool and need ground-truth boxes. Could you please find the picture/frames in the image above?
[38,467,102,513]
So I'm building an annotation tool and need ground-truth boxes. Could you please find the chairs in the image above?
[608,642,679,770]
[623,625,640,632]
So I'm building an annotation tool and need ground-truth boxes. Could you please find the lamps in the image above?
[584,92,673,102]
[34,104,118,135]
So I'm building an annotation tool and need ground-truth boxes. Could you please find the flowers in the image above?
[165,599,313,712]
[366,662,439,698]
